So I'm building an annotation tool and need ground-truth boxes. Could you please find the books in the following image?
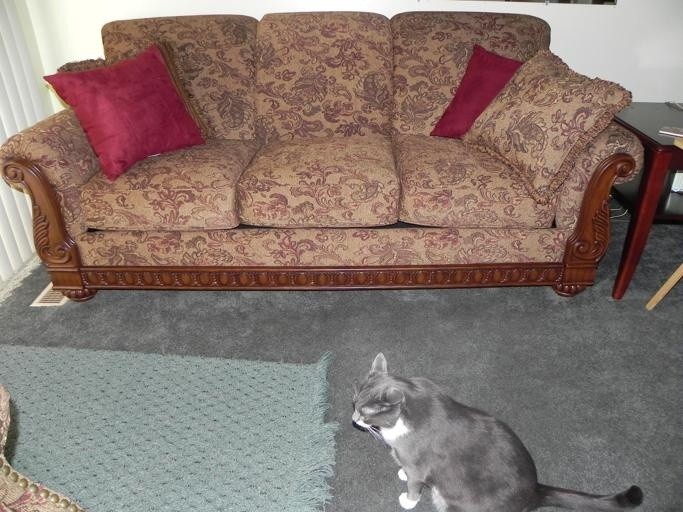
[658,125,682,138]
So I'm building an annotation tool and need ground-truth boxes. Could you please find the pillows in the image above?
[56,38,210,142]
[462,46,633,204]
[428,42,527,138]
[40,45,209,181]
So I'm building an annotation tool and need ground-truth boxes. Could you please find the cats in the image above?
[351,352,644,512]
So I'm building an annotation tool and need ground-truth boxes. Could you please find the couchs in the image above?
[2,9,644,296]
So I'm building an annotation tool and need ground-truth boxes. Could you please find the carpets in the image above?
[0,343,341,510]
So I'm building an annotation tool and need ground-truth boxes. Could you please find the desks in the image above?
[609,101,682,300]
